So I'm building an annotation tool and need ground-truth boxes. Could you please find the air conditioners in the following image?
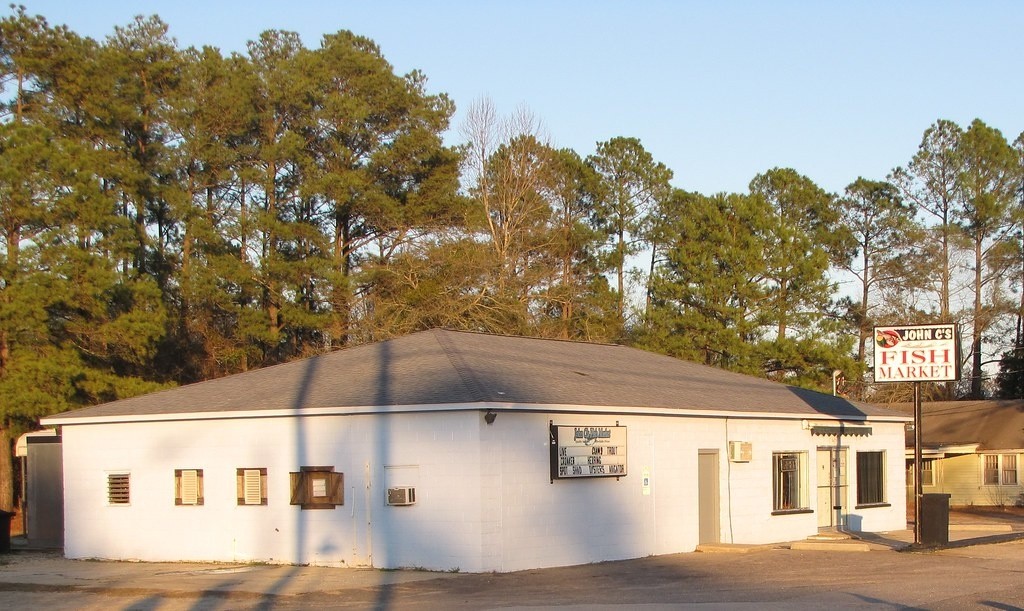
[728,442,753,462]
[386,486,415,505]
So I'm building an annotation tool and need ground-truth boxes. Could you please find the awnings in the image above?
[806,423,873,438]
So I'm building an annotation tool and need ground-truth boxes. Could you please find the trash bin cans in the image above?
[921,492,951,544]
[0,509,16,553]
